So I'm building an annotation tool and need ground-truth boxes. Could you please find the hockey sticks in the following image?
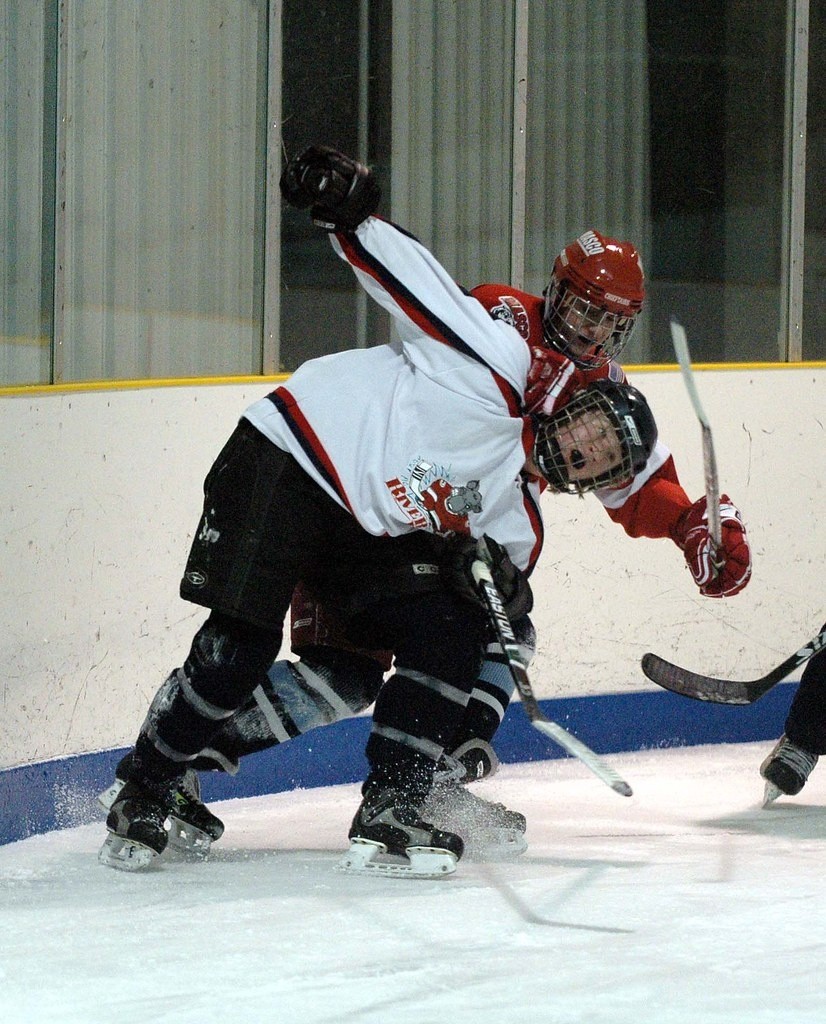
[667,313,722,543]
[467,553,639,795]
[642,628,825,706]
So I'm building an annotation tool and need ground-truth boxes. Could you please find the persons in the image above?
[100,230,753,864]
[759,622,826,805]
[100,142,658,880]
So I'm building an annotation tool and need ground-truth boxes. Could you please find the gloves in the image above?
[279,145,382,232]
[671,492,752,599]
[524,346,584,419]
[450,539,533,623]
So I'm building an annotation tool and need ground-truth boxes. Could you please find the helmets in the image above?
[531,381,658,495]
[542,230,644,374]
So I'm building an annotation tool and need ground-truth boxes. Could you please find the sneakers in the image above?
[417,739,529,857]
[97,782,169,872]
[759,734,818,808]
[334,787,464,879]
[95,747,240,857]
